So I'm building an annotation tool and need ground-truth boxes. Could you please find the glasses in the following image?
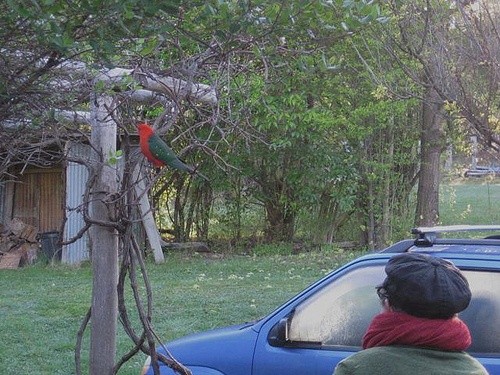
[377,286,392,305]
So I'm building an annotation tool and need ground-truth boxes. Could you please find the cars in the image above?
[142,226,500,375]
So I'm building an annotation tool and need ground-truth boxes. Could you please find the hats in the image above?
[383,253,472,319]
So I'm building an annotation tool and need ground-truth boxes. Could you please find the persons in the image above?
[333,253,489,375]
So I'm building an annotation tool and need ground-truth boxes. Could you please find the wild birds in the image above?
[136,123,208,180]
